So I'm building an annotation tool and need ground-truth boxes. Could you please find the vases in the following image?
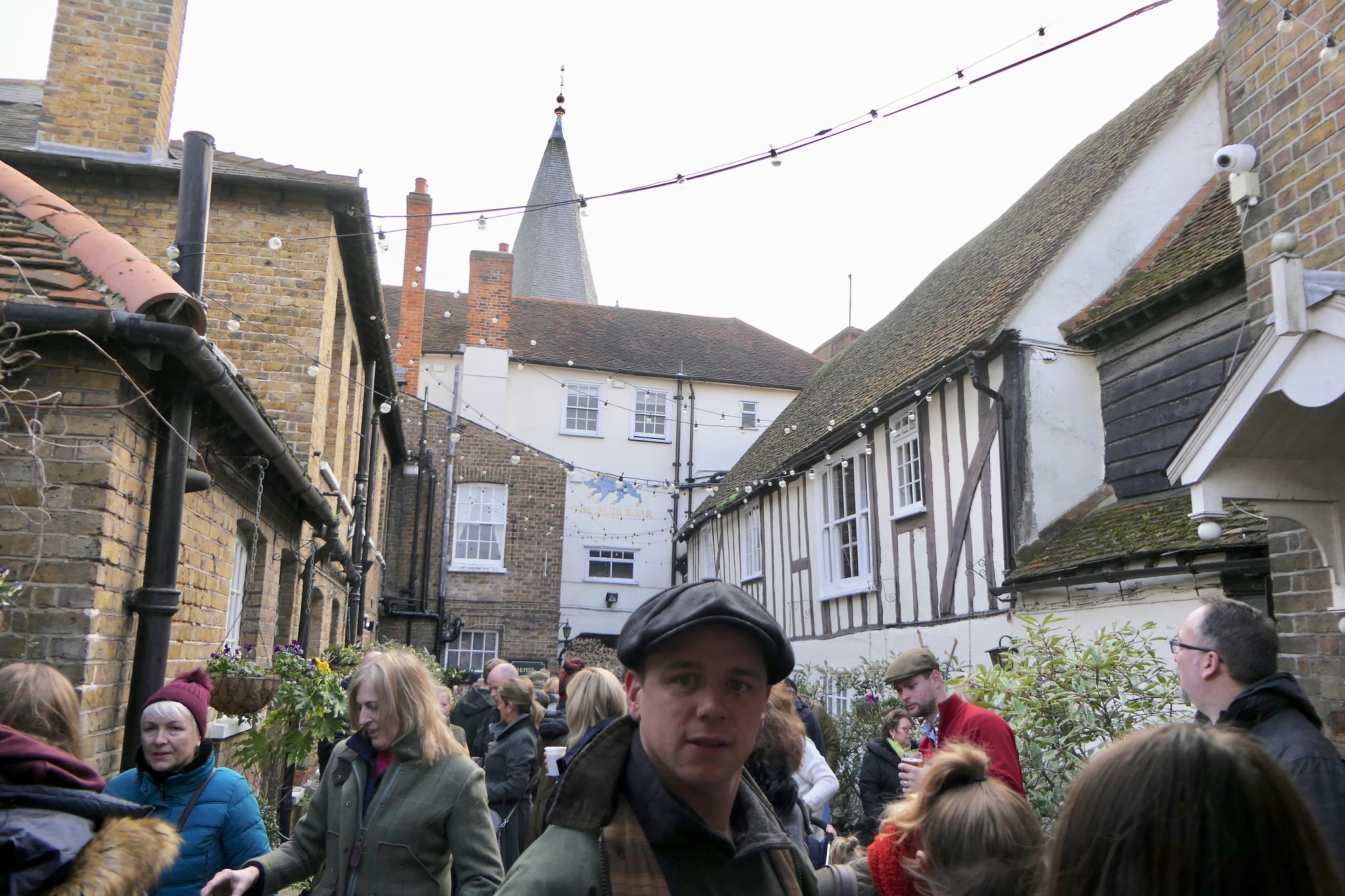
[206,674,281,715]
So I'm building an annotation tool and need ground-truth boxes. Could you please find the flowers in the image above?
[203,642,273,678]
[271,640,318,680]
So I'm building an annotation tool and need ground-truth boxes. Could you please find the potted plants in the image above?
[293,761,312,787]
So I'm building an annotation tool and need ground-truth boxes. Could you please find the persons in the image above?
[434,647,1028,896]
[494,576,824,896]
[1032,718,1345,896]
[198,648,505,896]
[1171,594,1345,896]
[317,651,384,783]
[100,668,280,896]
[815,735,1050,895]
[0,661,181,896]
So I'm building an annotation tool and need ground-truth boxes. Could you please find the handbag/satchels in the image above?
[486,803,503,841]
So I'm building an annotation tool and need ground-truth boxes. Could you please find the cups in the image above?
[901,749,923,794]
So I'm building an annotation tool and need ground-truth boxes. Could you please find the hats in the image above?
[615,580,798,685]
[528,671,549,682]
[883,646,940,687]
[141,666,214,741]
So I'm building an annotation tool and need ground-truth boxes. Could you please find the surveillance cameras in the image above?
[1214,143,1256,175]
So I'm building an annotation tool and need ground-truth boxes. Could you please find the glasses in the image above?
[894,725,915,732]
[1168,636,1225,667]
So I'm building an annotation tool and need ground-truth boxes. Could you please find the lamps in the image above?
[559,618,573,641]
[984,635,1018,672]
[363,613,375,632]
[606,592,618,608]
